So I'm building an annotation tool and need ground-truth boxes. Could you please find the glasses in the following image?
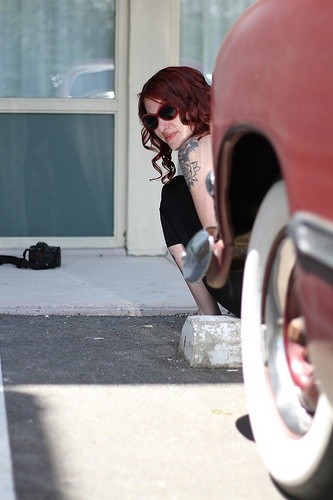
[141,101,179,132]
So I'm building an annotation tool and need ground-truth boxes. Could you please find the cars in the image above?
[212,0,333,500]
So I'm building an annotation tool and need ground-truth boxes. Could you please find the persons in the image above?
[138,66,246,317]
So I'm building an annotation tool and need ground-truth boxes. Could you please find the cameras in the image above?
[29,242,61,269]
